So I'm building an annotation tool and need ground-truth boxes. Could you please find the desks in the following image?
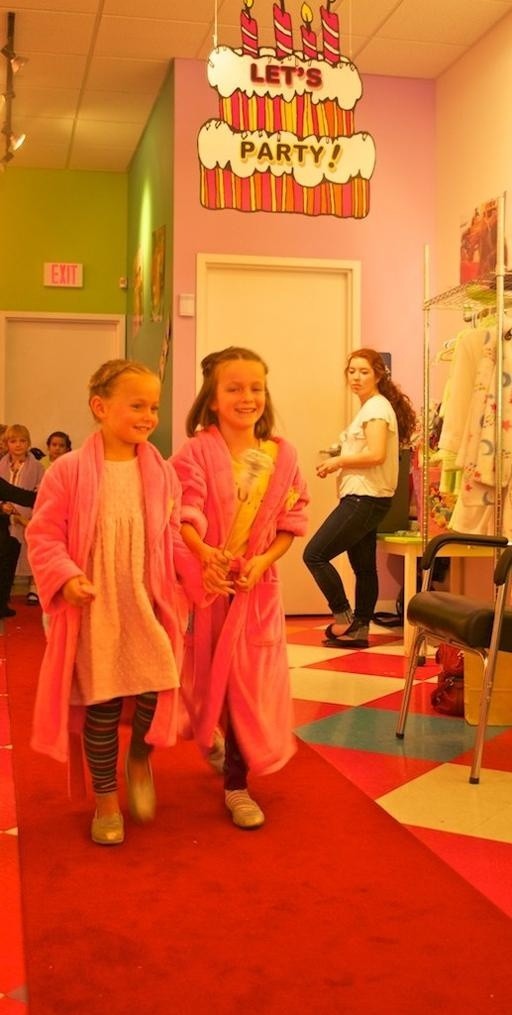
[374,533,511,661]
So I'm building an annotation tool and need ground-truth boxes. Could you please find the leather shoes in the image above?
[0,606,17,616]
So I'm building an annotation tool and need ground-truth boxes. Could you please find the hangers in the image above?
[432,302,504,367]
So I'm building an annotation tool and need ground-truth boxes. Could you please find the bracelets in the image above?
[337,456,343,467]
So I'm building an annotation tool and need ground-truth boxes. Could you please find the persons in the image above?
[166,342,311,834]
[302,344,419,647]
[40,428,75,477]
[26,360,236,846]
[0,422,43,606]
[0,423,9,456]
[0,476,41,622]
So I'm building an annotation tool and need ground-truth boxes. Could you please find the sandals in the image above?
[27,591,39,604]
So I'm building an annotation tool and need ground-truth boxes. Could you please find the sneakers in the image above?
[200,725,225,775]
[325,624,345,638]
[325,636,368,648]
[126,756,155,820]
[224,793,264,828]
[92,805,124,843]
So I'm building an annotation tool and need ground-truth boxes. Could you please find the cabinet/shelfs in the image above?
[418,188,511,566]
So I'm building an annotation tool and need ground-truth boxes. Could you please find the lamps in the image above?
[2,11,29,166]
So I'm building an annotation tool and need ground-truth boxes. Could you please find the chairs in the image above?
[395,527,512,788]
